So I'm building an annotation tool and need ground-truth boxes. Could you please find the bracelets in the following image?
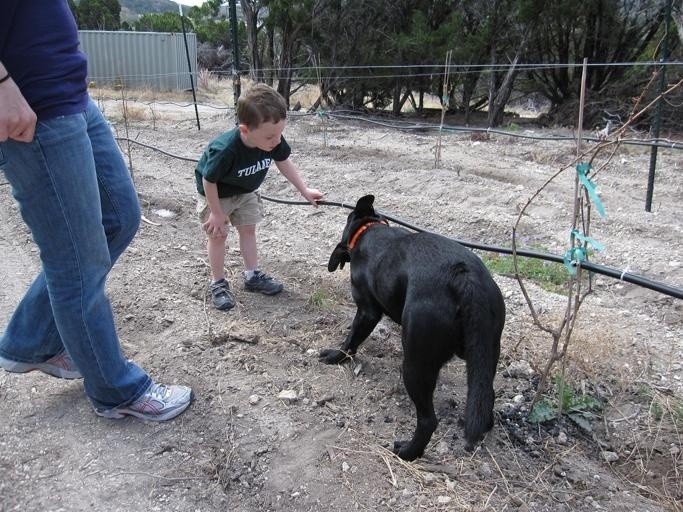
[0,73,12,85]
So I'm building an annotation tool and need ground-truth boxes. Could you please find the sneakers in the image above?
[85,382,193,422]
[243,273,283,295]
[210,279,236,310]
[0,347,83,380]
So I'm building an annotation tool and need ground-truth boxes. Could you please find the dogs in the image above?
[317,194,506,462]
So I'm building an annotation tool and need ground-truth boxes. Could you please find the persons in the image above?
[192,82,326,310]
[0,1,196,425]
